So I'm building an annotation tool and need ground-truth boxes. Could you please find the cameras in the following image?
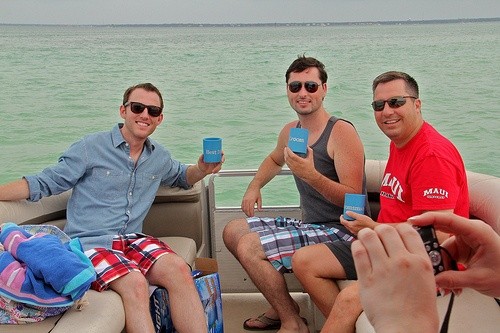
[412,225,445,276]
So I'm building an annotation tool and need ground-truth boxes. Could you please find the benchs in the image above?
[306,160,500,333]
[0,183,211,333]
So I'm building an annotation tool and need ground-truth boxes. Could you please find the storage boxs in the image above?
[149,258,224,333]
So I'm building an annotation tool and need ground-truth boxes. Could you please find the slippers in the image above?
[243,312,308,331]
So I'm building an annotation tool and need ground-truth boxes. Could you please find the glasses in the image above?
[124,101,162,117]
[287,81,324,93]
[370,96,417,112]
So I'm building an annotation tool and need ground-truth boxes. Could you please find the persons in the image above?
[352,212,500,333]
[0,83,225,333]
[292,71,470,333]
[224,57,371,333]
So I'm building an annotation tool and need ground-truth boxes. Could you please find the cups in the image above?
[344,194,366,220]
[203,138,222,163]
[288,128,308,155]
